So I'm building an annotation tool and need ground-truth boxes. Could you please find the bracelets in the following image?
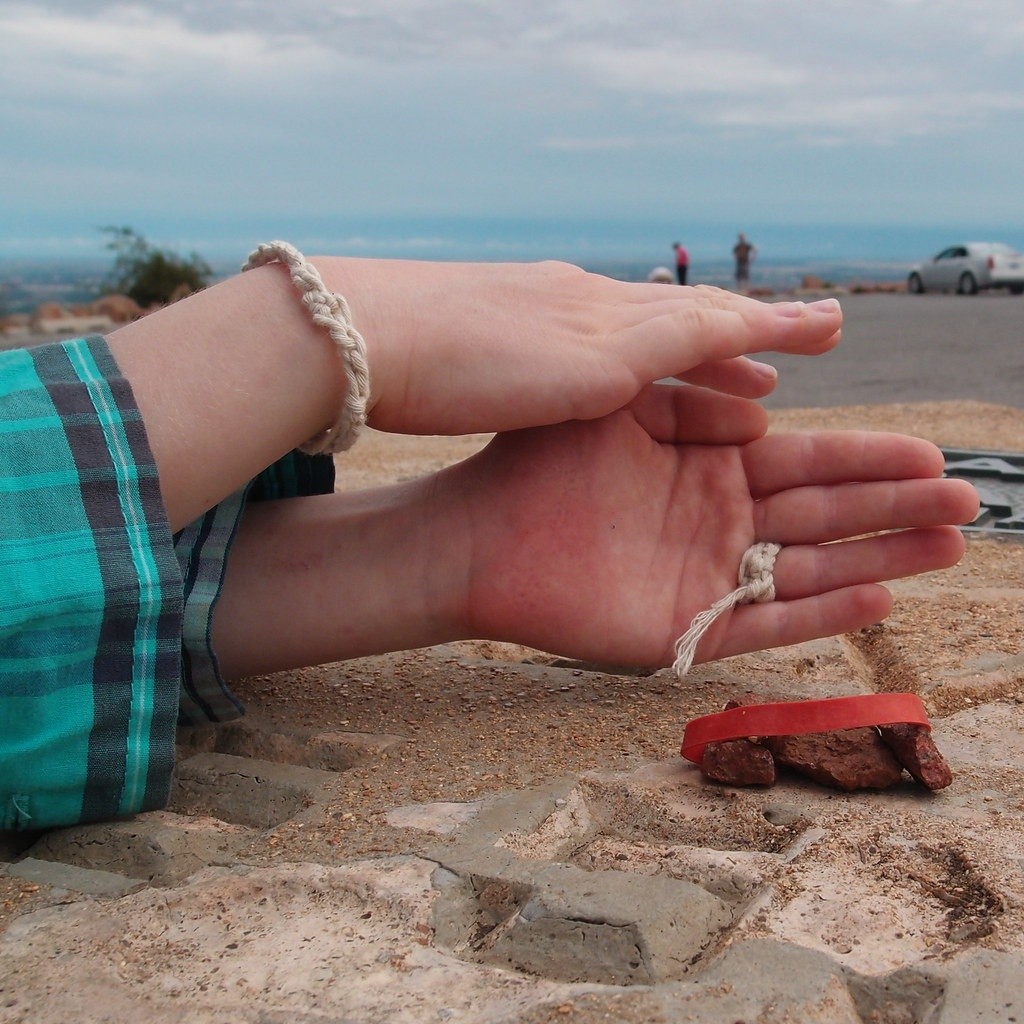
[239,242,369,456]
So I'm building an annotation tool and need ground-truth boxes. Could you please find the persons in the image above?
[733,236,755,294]
[671,245,689,286]
[1,241,977,860]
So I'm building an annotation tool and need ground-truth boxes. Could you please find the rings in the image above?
[737,542,778,607]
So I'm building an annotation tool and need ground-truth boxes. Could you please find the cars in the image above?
[908,242,1024,297]
